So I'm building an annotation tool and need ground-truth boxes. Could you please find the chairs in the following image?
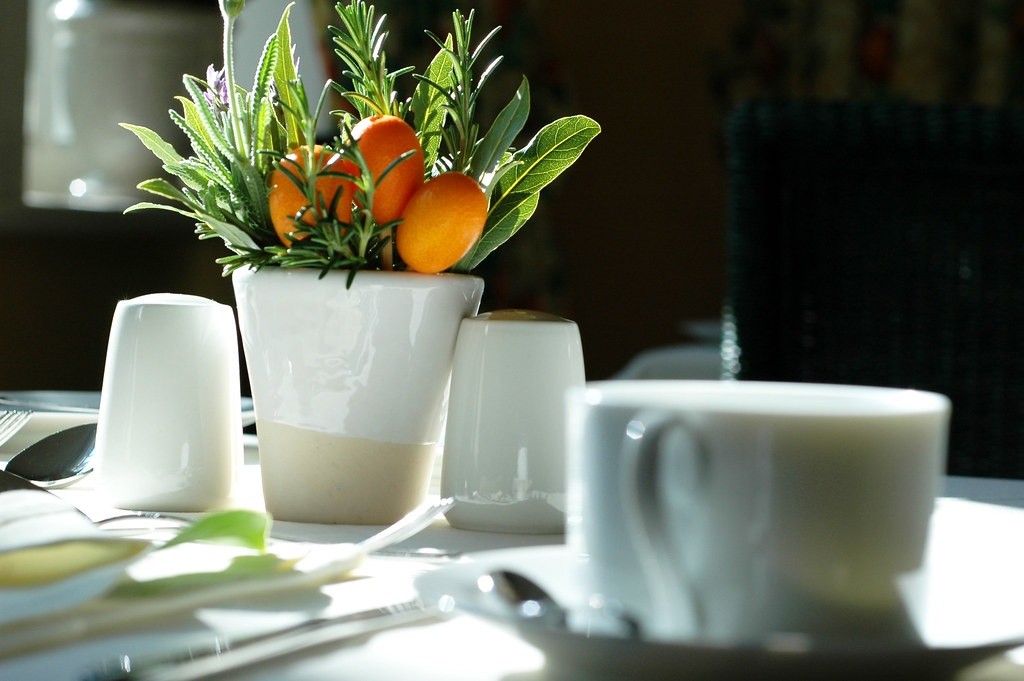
[721,97,1024,483]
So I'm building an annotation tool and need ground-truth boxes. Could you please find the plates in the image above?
[415,557,1024,681]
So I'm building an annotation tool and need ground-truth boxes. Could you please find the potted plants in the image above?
[118,1,602,523]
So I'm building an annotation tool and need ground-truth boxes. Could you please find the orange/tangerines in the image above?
[269,115,486,274]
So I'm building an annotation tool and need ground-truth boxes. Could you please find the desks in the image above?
[1,391,1024,681]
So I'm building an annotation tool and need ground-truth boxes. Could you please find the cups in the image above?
[441,309,586,533]
[568,379,951,645]
[94,293,245,512]
